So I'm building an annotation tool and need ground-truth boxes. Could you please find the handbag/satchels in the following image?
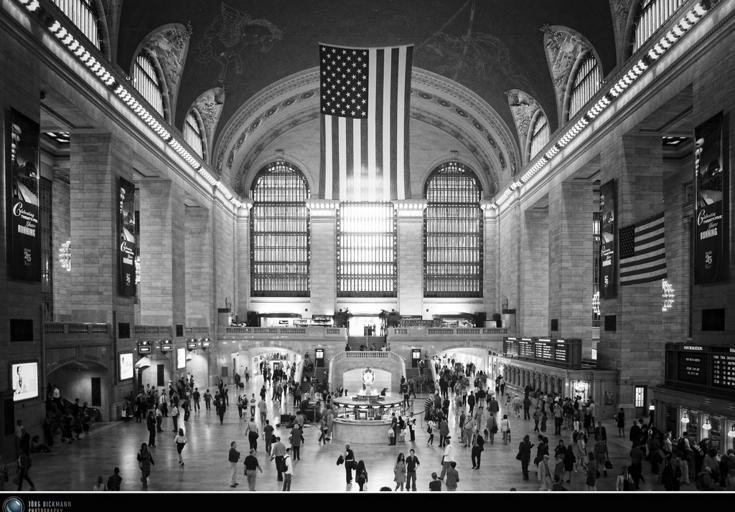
[586,477,595,486]
[354,461,357,469]
[427,426,432,433]
[492,426,497,432]
[605,460,612,468]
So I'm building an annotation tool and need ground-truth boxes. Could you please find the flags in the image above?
[317,42,414,202]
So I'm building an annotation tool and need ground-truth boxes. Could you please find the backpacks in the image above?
[278,456,289,472]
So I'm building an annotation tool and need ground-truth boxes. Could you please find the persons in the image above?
[13,344,735,491]
